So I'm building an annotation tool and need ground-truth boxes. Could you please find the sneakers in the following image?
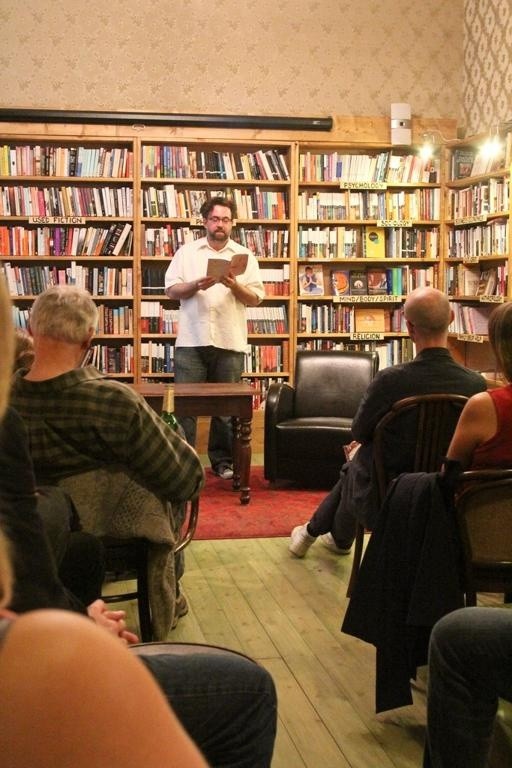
[289,521,318,557]
[172,593,189,629]
[219,468,234,478]
[322,534,351,554]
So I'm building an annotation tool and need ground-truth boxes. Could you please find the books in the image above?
[296,148,440,371]
[141,145,291,410]
[444,131,512,335]
[0,143,134,375]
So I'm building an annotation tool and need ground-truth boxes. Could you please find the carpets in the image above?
[179,461,333,540]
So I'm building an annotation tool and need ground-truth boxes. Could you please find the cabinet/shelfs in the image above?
[443,130,511,384]
[293,134,444,391]
[136,139,294,385]
[1,129,138,380]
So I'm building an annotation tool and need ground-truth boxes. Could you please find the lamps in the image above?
[418,131,442,160]
[478,129,505,162]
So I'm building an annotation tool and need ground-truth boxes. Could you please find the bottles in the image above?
[158,382,180,433]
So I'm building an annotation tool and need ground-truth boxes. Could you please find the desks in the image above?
[131,382,259,504]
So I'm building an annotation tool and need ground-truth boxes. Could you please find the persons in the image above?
[288,286,487,558]
[0,405,109,607]
[163,196,267,480]
[422,602,511,767]
[13,283,206,629]
[0,523,278,768]
[439,301,512,475]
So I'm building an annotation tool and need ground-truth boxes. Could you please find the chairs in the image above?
[385,469,512,693]
[267,350,379,490]
[36,486,202,644]
[347,395,467,599]
